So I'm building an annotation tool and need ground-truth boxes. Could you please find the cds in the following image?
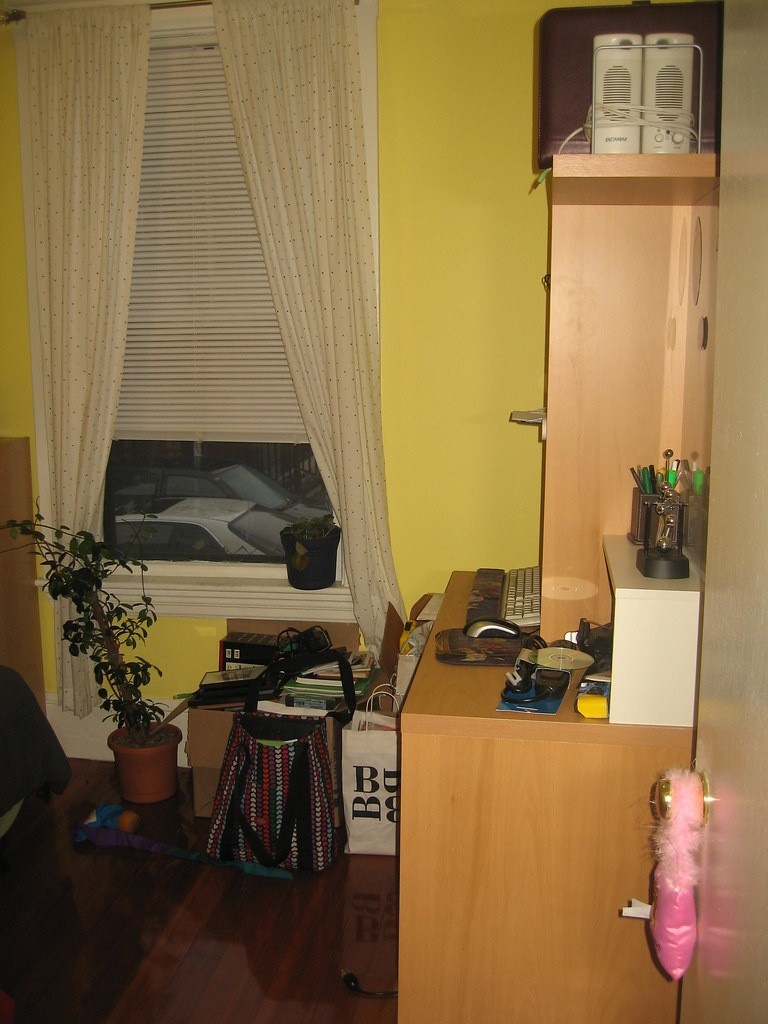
[542,577,598,601]
[527,647,595,670]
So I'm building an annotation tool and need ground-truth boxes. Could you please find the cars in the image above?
[108,451,331,563]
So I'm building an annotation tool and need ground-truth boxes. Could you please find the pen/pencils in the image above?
[630,459,683,495]
[680,457,710,496]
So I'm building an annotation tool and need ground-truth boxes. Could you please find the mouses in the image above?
[463,616,520,638]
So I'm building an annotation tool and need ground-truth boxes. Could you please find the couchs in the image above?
[0,664,72,873]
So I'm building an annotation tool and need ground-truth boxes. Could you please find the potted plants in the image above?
[280,514,342,590]
[0,496,183,803]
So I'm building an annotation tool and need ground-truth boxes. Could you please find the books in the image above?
[254,651,377,718]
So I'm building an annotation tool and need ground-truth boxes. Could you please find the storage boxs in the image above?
[148,593,446,828]
[538,0,723,172]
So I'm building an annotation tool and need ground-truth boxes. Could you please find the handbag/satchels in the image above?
[341,683,403,856]
[205,649,357,873]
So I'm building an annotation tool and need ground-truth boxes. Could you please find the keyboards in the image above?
[500,565,541,628]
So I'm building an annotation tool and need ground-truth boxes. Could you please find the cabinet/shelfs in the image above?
[398,153,719,1023]
